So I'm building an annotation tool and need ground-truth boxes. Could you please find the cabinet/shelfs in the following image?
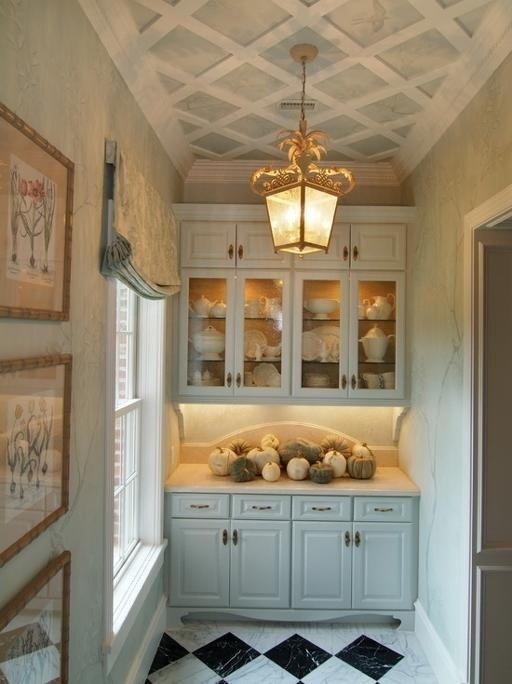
[292,271,407,402]
[166,493,292,612]
[178,268,291,398]
[179,218,292,269]
[293,220,408,271]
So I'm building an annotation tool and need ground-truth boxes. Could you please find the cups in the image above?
[245,371,252,385]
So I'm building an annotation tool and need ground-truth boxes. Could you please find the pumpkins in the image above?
[207,434,376,482]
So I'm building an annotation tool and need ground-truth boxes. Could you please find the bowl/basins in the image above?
[364,372,394,389]
[304,374,330,386]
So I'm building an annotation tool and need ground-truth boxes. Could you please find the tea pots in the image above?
[318,341,332,363]
[187,369,214,384]
[361,292,396,318]
[189,294,225,320]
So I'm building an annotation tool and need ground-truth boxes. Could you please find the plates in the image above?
[242,329,266,357]
[312,325,339,362]
[300,332,323,362]
[253,363,280,387]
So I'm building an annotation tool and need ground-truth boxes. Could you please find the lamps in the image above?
[248,42,356,259]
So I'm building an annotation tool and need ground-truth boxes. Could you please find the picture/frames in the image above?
[0,354,75,568]
[292,495,421,613]
[0,100,74,322]
[0,550,70,684]
